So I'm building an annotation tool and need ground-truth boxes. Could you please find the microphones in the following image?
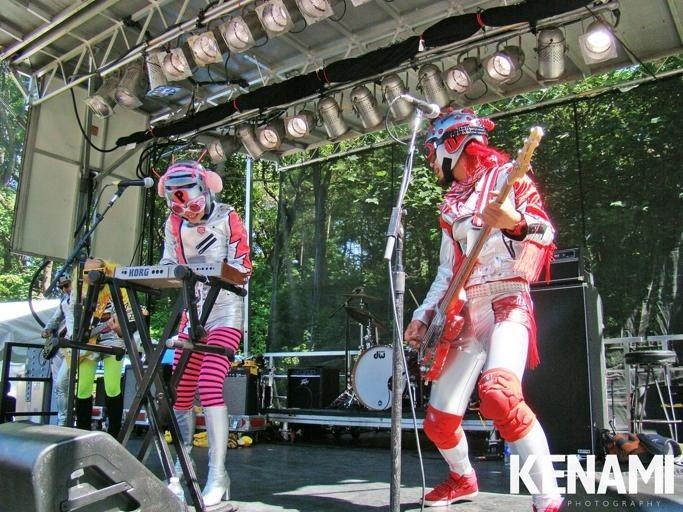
[110,176,155,192]
[399,92,442,120]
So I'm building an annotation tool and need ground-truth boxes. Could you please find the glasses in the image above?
[58,282,68,289]
[167,194,207,215]
[422,138,445,161]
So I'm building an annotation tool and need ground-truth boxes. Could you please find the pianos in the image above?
[113,262,245,289]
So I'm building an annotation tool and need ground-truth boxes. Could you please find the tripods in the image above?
[331,322,362,410]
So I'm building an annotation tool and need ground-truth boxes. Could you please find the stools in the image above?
[626,349,678,443]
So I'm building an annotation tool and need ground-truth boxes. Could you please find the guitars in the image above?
[417,126,544,382]
[42,305,149,368]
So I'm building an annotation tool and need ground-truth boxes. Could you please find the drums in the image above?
[352,345,419,412]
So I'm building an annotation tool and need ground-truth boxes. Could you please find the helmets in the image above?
[158,159,223,215]
[421,106,495,184]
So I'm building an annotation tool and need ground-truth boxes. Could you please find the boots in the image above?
[174,407,198,487]
[201,405,231,507]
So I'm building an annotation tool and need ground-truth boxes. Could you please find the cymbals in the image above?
[341,292,384,304]
[344,306,386,334]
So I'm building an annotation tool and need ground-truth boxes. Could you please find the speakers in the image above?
[501,283,610,469]
[222,372,259,415]
[0,419,193,512]
[286,367,340,411]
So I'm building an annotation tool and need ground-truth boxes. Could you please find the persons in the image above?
[41,263,74,426]
[75,256,138,441]
[404,106,564,512]
[151,161,253,507]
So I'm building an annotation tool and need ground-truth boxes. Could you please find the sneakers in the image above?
[418,468,479,508]
